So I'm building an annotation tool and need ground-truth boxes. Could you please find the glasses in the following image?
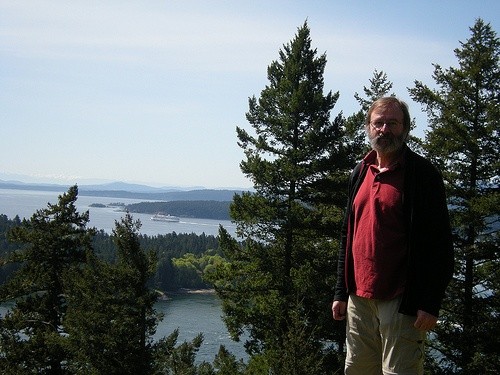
[369,120,405,128]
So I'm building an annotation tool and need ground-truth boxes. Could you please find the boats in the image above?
[150,212,179,222]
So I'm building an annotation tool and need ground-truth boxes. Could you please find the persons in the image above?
[330,96,446,375]
